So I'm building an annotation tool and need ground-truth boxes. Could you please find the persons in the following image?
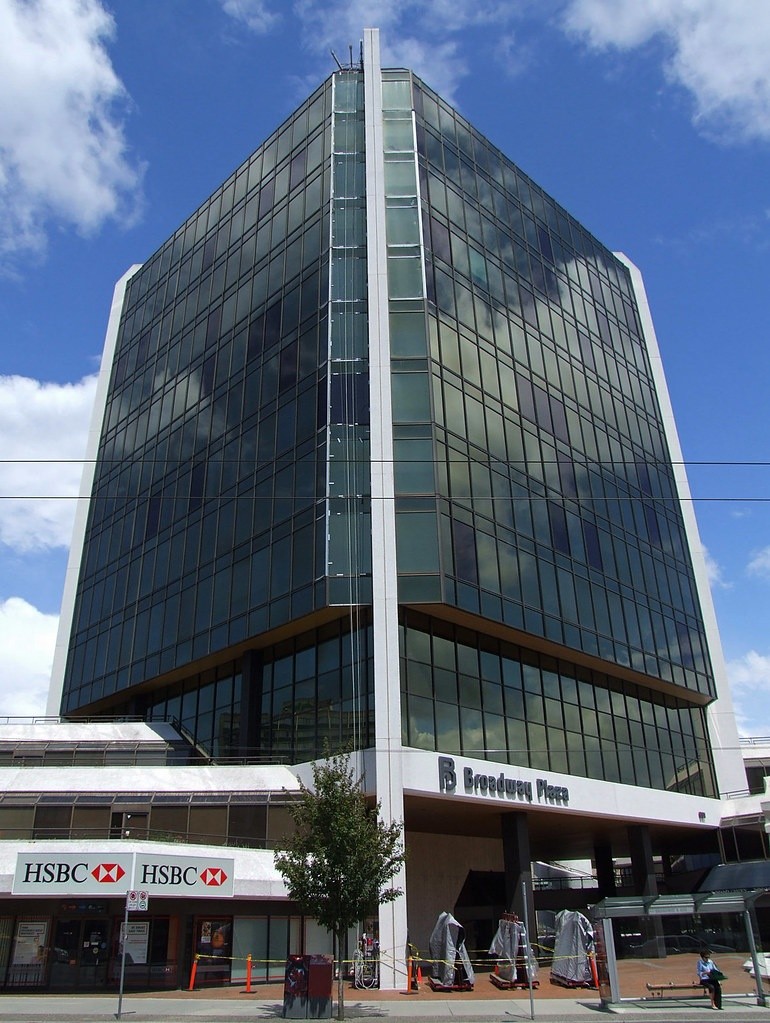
[696,950,720,1009]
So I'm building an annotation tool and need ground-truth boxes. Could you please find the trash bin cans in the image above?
[282,953,335,1019]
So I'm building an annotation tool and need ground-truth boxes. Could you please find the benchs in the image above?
[646,982,707,999]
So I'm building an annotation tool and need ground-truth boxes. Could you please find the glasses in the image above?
[703,956,709,958]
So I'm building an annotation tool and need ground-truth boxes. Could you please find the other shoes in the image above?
[712,1005,718,1009]
